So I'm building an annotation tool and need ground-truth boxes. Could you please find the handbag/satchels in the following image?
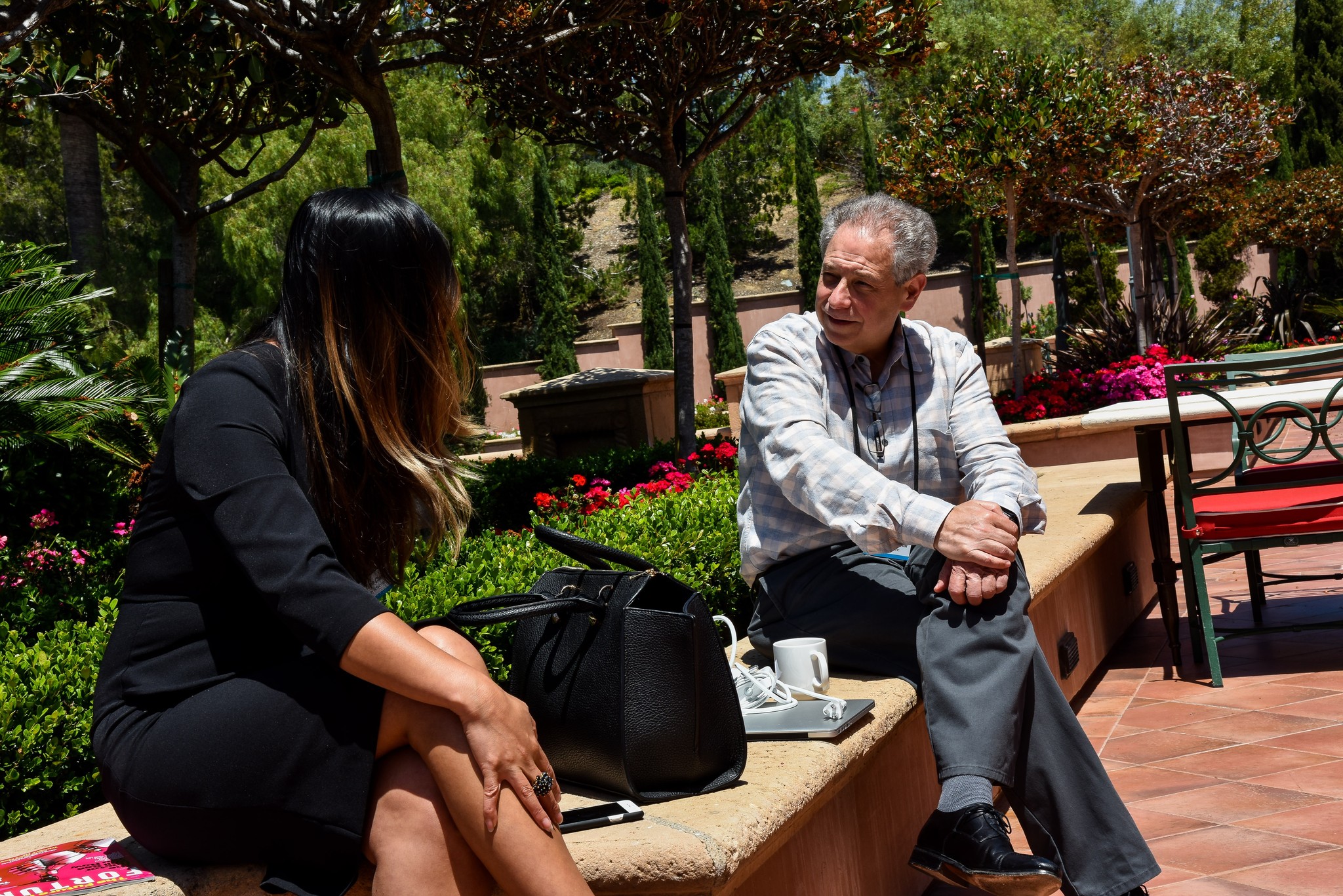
[447,522,747,806]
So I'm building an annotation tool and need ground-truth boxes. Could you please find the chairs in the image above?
[1152,341,1343,690]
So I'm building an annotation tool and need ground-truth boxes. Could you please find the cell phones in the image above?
[559,800,644,835]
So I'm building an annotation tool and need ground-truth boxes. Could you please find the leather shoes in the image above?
[908,802,1063,896]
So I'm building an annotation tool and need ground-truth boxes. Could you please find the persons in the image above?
[739,193,1163,896]
[85,187,599,896]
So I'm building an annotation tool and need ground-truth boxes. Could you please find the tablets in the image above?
[741,699,876,740]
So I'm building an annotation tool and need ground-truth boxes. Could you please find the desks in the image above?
[1079,375,1343,668]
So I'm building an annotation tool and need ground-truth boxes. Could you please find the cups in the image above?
[773,637,829,701]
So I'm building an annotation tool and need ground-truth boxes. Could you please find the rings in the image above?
[531,772,554,798]
